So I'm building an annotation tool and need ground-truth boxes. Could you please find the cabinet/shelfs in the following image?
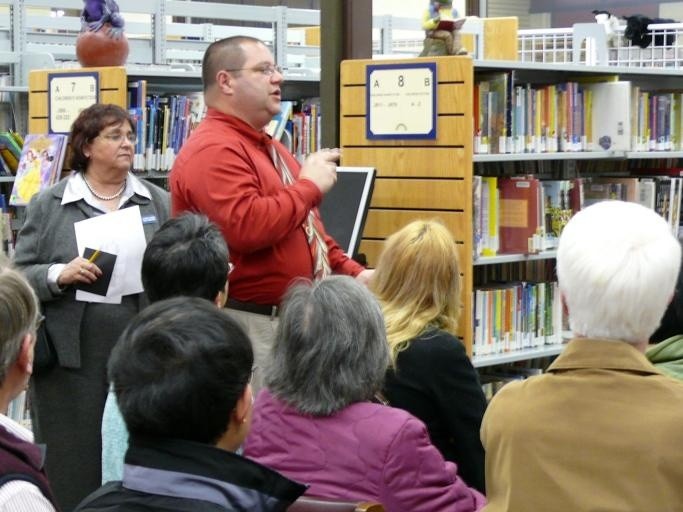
[1,1,320,432]
[28,67,321,191]
[339,56,683,404]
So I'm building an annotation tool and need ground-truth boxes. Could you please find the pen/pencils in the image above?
[88,247,102,263]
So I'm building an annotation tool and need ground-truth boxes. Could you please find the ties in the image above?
[266,141,332,283]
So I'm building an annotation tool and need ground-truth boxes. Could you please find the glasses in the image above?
[226,63,281,75]
[102,130,138,144]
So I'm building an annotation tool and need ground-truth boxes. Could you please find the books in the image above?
[432,18,466,33]
[474,257,574,403]
[1,88,323,261]
[478,16,520,62]
[473,161,683,261]
[473,66,683,154]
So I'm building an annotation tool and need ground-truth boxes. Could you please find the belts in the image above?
[224,294,281,317]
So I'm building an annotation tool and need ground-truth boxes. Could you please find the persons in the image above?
[9,103,174,512]
[1,212,494,512]
[168,34,373,409]
[421,1,467,57]
[479,199,683,512]
